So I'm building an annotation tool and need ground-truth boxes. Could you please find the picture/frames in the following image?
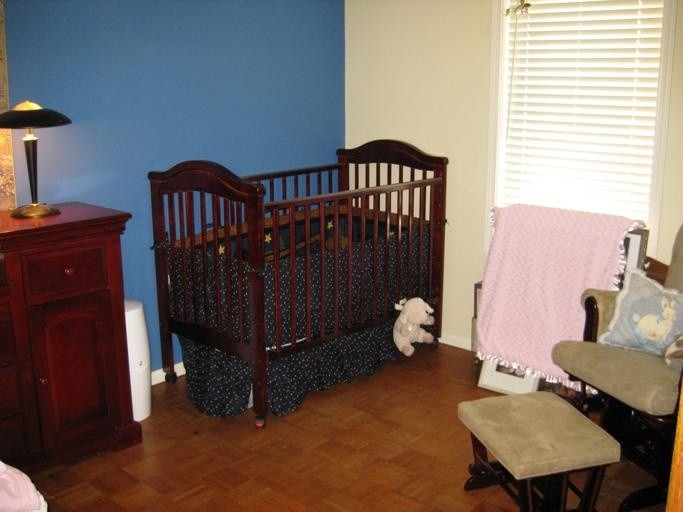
[616,228,650,292]
[477,360,542,395]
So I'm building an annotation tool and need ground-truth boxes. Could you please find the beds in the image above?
[147,138,449,428]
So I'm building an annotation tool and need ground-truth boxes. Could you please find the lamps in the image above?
[0,100,72,219]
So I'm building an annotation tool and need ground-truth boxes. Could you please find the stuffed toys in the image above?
[391,296,437,356]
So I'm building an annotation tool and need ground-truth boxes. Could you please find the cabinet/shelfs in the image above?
[1,201,144,477]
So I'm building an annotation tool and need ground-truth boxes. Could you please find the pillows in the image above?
[597,267,683,359]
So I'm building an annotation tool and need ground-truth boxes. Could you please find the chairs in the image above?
[550,223,683,512]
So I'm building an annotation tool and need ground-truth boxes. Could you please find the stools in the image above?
[457,390,621,511]
